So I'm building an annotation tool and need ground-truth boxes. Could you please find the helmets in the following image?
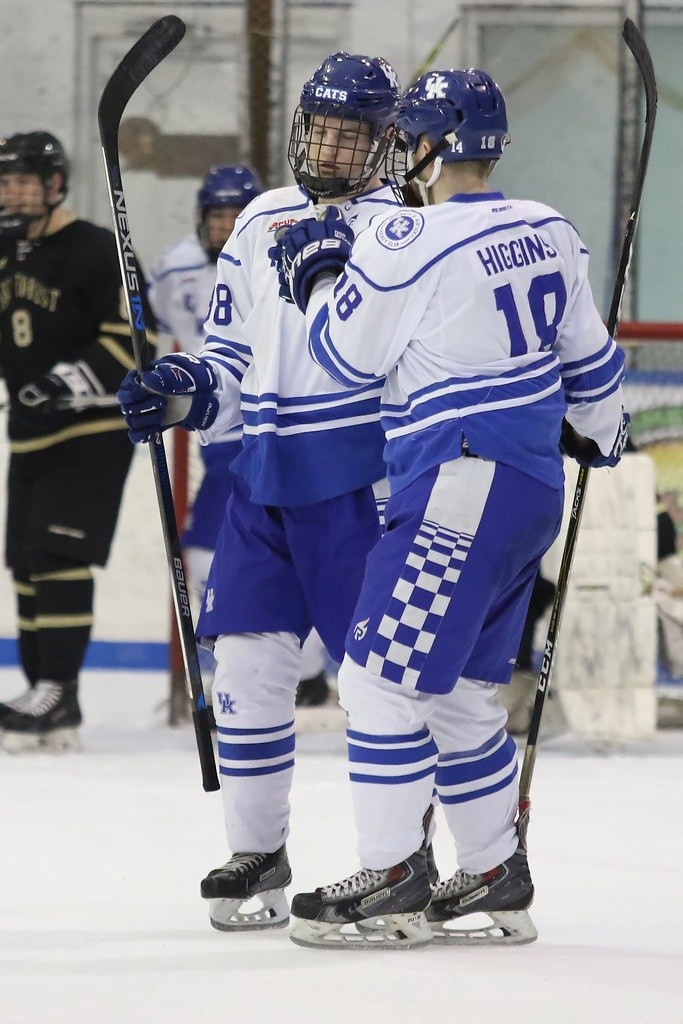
[385,68,512,206]
[285,49,401,201]
[193,164,265,263]
[0,131,69,220]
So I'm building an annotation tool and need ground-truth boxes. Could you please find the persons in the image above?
[278,66,627,948]
[0,52,440,930]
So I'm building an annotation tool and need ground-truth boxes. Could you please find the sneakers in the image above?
[289,803,442,948]
[200,841,292,931]
[0,678,82,751]
[424,794,539,945]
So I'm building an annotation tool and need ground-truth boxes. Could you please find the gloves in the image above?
[268,205,355,315]
[559,414,631,470]
[117,351,219,445]
[9,360,106,437]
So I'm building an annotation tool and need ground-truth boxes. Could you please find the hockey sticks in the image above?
[92,10,225,797]
[517,17,661,804]
[1,394,122,414]
